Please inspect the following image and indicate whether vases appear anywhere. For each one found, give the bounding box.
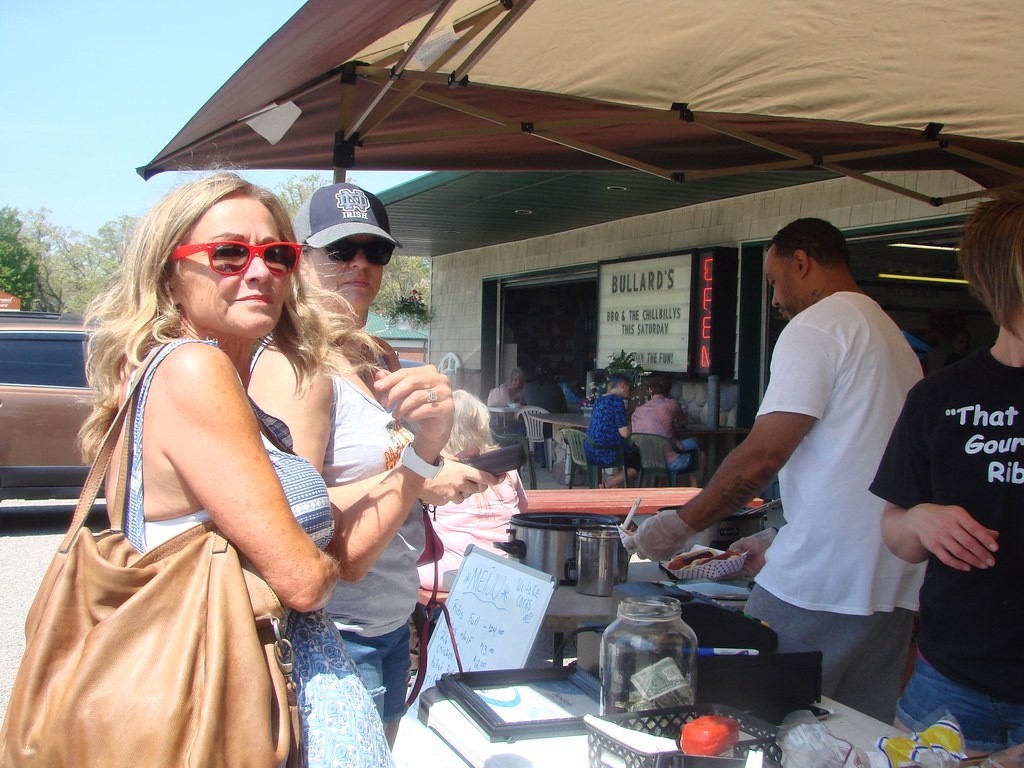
[581,407,593,417]
[397,314,419,330]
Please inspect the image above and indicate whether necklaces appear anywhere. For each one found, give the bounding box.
[330,339,369,381]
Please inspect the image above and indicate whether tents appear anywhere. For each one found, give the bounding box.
[133,0,1024,207]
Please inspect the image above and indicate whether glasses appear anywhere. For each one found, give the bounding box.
[168,241,303,276]
[324,238,395,265]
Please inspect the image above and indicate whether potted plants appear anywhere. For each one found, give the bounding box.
[603,349,651,410]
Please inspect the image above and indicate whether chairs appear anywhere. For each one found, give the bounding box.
[513,406,563,472]
[625,434,702,488]
[490,426,536,490]
[560,428,629,490]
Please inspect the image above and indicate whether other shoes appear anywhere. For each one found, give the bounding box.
[540,461,553,468]
[598,483,605,489]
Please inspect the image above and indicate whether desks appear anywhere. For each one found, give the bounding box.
[529,412,750,487]
[485,406,522,433]
[522,487,763,516]
[545,561,761,668]
[418,669,980,768]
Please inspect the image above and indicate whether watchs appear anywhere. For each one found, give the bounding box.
[401,441,445,479]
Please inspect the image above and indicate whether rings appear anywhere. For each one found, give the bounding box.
[423,387,437,402]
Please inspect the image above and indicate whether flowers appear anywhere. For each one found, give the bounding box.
[581,384,605,407]
[388,288,434,328]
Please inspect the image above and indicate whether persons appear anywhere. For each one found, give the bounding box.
[1,169,455,768]
[867,192,1024,757]
[245,185,507,753]
[584,372,641,490]
[631,371,706,488]
[489,367,567,466]
[623,217,925,729]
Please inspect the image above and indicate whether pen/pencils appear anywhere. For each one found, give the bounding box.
[683,646,759,655]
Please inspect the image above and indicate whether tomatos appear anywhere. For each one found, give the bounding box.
[680,715,739,756]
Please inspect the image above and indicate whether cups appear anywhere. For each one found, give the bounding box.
[576,525,620,595]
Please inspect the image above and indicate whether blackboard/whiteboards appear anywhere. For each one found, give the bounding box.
[405,542,560,713]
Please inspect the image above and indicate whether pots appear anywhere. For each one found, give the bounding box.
[657,503,767,552]
[493,512,630,585]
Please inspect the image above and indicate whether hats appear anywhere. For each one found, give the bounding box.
[293,182,403,249]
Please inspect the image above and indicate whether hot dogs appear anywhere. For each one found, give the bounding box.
[669,548,743,569]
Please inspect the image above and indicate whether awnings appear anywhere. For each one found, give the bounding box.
[371,166,869,256]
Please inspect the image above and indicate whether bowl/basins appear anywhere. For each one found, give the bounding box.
[508,403,520,408]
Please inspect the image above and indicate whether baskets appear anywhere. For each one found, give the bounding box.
[583,702,783,768]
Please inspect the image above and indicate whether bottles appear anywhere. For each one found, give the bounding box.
[680,716,739,759]
[599,596,697,716]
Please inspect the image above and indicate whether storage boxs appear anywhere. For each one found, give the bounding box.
[583,703,782,768]
[661,545,750,578]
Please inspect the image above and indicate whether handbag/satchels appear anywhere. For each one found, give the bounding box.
[415,498,444,567]
[0,340,302,768]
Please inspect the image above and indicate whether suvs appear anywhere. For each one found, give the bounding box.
[0,311,107,513]
